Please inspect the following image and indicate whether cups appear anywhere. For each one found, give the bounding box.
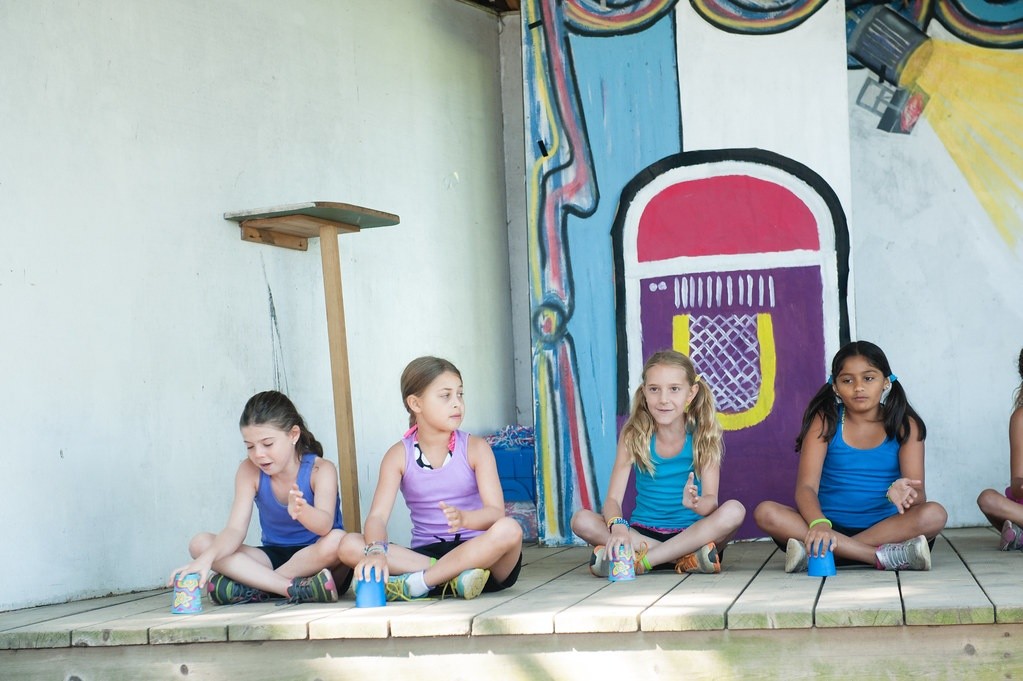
[172,576,203,613]
[355,567,386,608]
[608,546,635,581]
[806,540,836,577]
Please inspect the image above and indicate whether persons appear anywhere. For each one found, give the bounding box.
[753,341,948,572]
[571,350,746,577]
[336,355,523,601]
[977,346,1023,551]
[169,389,354,605]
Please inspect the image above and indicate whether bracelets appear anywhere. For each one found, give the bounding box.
[364,541,388,555]
[809,518,832,529]
[607,516,629,533]
[885,480,897,503]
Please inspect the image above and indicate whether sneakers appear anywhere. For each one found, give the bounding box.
[275,569,337,603]
[351,569,430,603]
[999,519,1023,552]
[875,536,932,571]
[784,537,809,573]
[676,542,722,574]
[206,570,271,605]
[590,545,649,578]
[447,569,491,600]
